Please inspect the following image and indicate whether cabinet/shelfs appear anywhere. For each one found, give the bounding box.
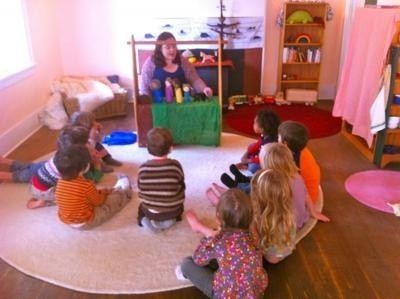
[276,2,328,101]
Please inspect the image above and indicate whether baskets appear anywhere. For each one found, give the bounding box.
[62,90,128,120]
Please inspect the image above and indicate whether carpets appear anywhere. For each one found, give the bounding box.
[223,103,342,139]
[0,127,326,294]
[344,170,400,215]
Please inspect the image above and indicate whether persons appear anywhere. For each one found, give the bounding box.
[204,142,330,231]
[140,31,214,99]
[136,127,186,235]
[93,142,124,174]
[182,166,299,267]
[234,109,282,174]
[50,143,133,232]
[24,124,92,209]
[0,110,105,184]
[174,188,270,299]
[219,119,322,206]
